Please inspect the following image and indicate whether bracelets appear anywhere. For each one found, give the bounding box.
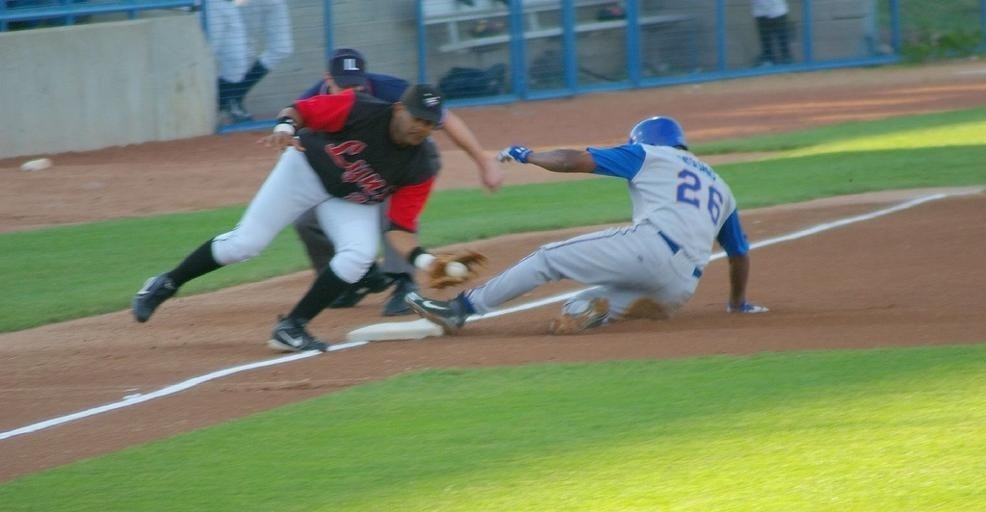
[409,246,426,265]
[272,124,294,136]
[415,254,436,271]
[273,115,296,130]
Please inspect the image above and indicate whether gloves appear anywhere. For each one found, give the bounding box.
[497,143,533,164]
[727,301,771,315]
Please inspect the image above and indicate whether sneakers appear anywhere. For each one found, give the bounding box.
[382,279,419,317]
[269,314,329,354]
[547,295,610,336]
[131,271,179,323]
[222,97,252,123]
[404,290,469,337]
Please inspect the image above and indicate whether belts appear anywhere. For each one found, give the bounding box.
[657,231,704,279]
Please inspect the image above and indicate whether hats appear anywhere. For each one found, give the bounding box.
[328,47,368,89]
[400,83,443,126]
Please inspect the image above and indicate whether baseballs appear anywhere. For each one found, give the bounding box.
[446,261,467,276]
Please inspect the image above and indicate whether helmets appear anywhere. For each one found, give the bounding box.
[628,115,689,151]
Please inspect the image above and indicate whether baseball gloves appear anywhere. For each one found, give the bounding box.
[430,249,486,288]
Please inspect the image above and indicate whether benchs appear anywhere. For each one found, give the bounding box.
[419,1,692,69]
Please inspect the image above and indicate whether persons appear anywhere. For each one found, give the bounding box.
[131,84,443,355]
[749,0,792,69]
[296,47,501,317]
[202,1,293,126]
[405,117,771,338]
